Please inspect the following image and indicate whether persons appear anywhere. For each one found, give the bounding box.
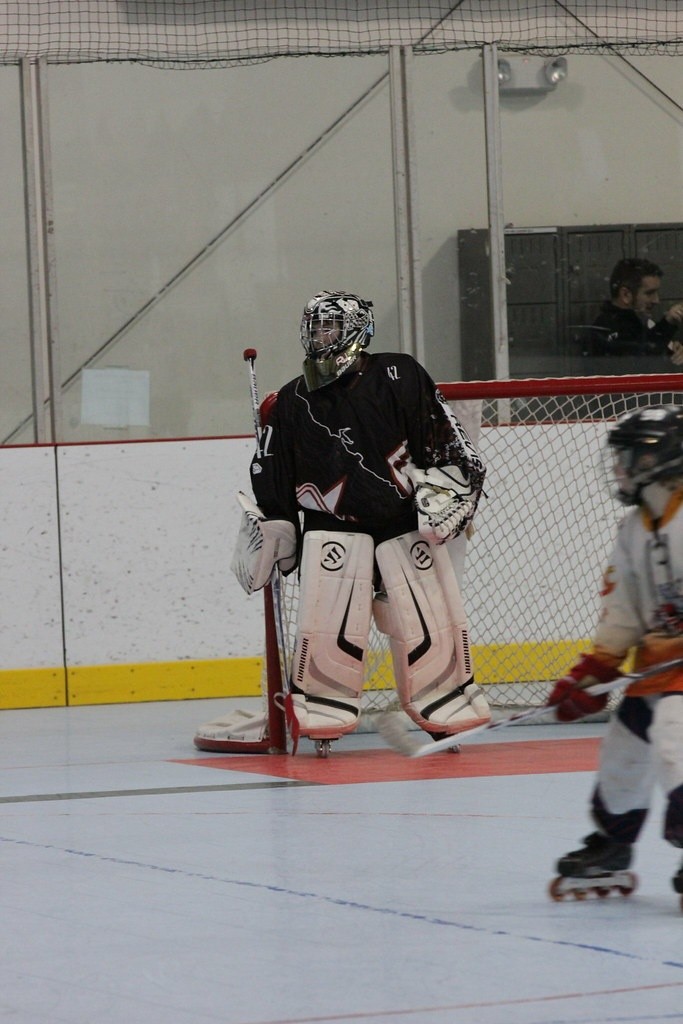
[548,403,683,891]
[578,257,682,375]
[249,290,491,736]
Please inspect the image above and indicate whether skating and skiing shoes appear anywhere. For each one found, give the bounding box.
[428,730,460,754]
[549,834,639,900]
[311,737,335,757]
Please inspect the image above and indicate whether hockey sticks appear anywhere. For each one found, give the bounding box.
[243,348,300,757]
[374,656,683,760]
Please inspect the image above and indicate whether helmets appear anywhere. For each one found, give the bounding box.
[299,290,375,391]
[607,404,683,506]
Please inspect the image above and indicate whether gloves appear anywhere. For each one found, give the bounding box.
[547,653,618,723]
